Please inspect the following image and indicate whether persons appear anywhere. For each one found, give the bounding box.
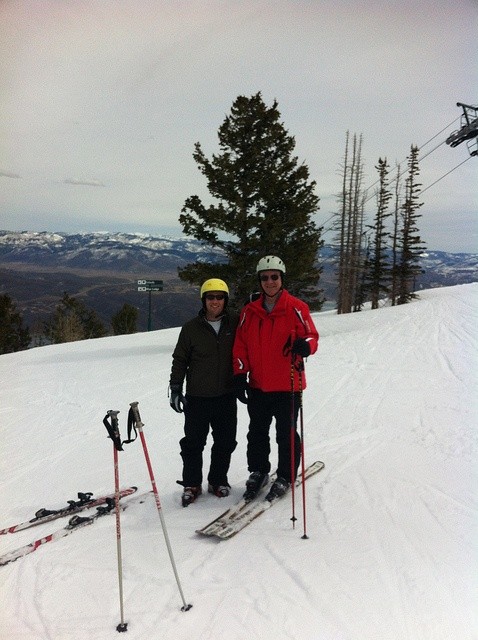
[169,278,245,506]
[233,256,318,494]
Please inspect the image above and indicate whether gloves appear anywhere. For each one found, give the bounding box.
[290,338,312,358]
[232,373,251,403]
[168,384,188,413]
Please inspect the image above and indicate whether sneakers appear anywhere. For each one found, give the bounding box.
[270,478,289,498]
[182,485,201,507]
[246,472,269,491]
[208,482,229,497]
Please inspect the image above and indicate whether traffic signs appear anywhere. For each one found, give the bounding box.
[137,286,163,292]
[137,279,163,285]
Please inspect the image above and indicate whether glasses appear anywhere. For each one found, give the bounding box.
[261,274,278,282]
[205,295,224,300]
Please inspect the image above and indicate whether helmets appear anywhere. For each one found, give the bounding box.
[255,255,286,286]
[200,278,230,300]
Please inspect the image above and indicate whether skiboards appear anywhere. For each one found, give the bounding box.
[195,461,325,541]
[0,486,156,567]
